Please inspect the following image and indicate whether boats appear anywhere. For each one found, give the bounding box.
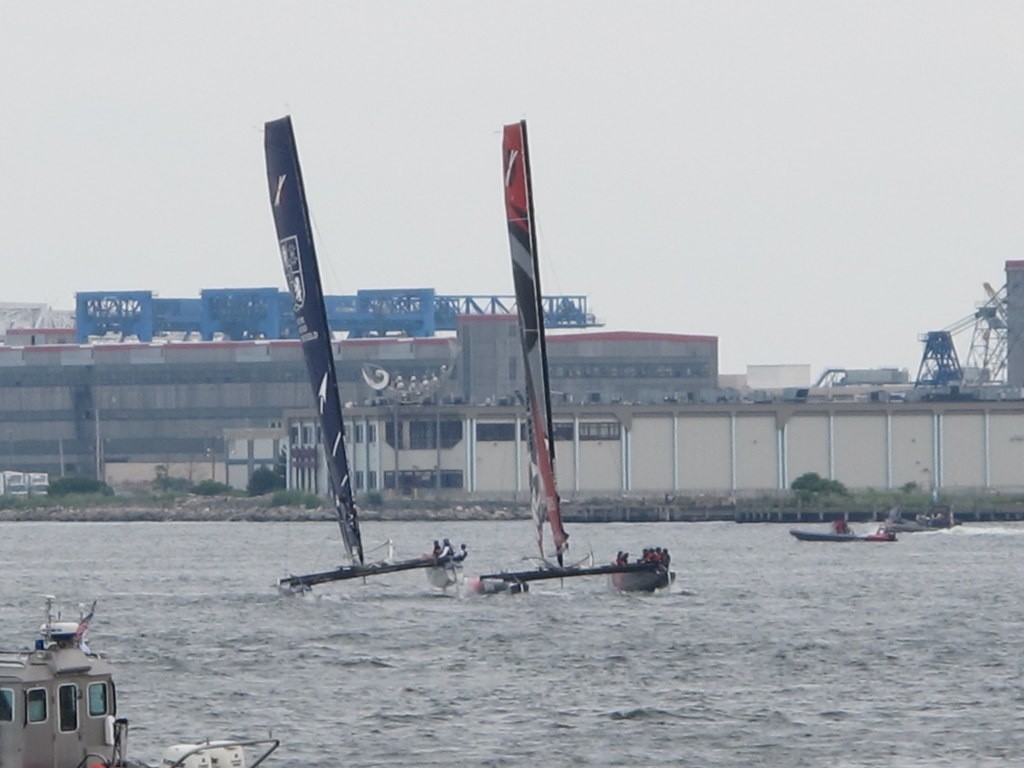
[788,521,899,542]
[0,595,280,768]
[883,504,962,533]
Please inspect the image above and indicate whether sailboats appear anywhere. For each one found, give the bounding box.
[261,116,466,600]
[465,117,678,597]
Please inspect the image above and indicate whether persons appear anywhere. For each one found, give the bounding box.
[918,511,944,521]
[636,548,670,570]
[615,551,629,567]
[832,517,849,534]
[431,538,467,562]
